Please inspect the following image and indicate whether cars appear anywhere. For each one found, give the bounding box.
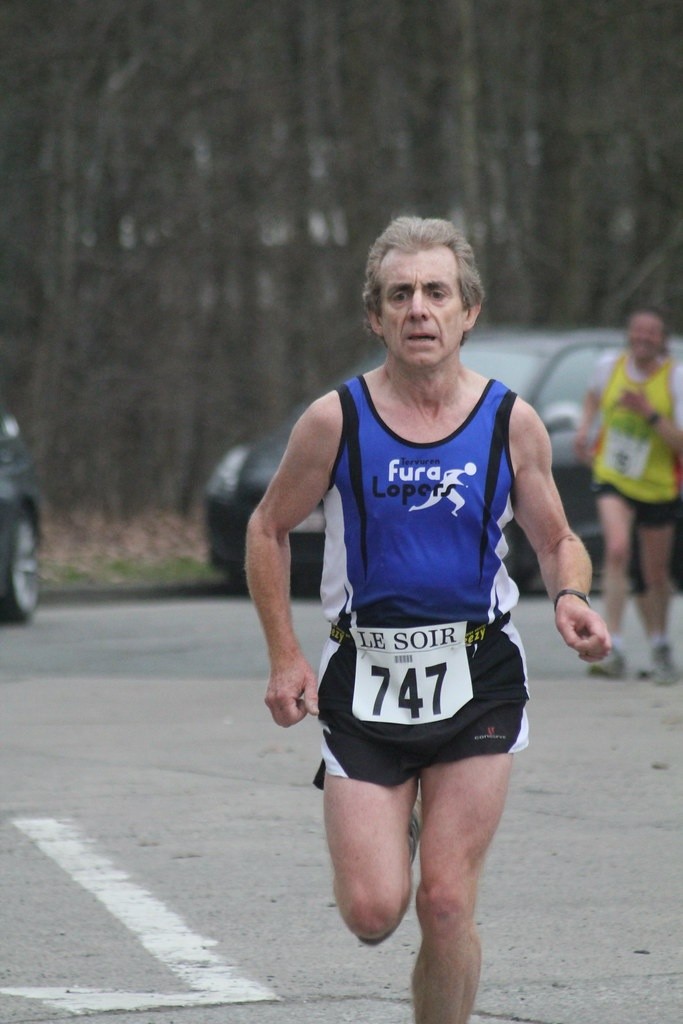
[0,408,43,623]
[200,327,683,607]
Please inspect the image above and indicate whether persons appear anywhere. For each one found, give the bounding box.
[245,212,613,1024]
[575,306,683,684]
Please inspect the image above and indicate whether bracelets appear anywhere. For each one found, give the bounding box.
[647,415,658,425]
[554,589,590,610]
